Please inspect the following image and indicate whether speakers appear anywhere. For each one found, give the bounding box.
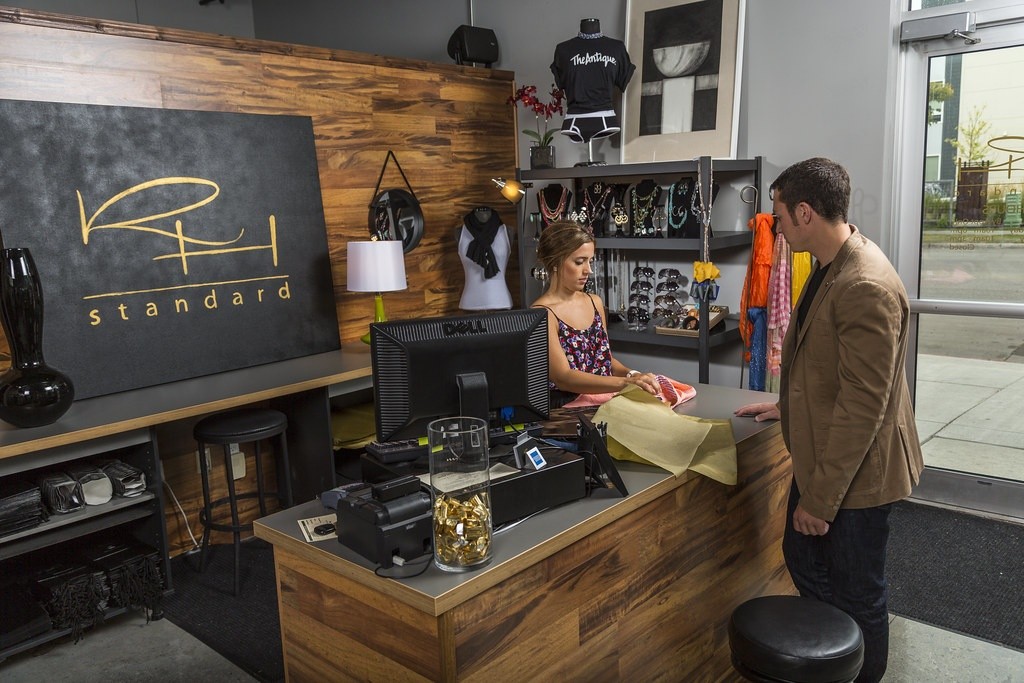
[447,24,498,64]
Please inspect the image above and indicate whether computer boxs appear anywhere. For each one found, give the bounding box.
[360,439,587,525]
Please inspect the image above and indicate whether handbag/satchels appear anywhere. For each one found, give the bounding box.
[0,476,42,537]
[0,532,167,650]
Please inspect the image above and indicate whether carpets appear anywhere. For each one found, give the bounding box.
[152,537,284,683]
[883,499,1023,651]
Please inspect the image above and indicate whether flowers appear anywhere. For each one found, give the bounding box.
[505,83,568,146]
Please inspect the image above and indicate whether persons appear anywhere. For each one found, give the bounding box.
[733,158,925,683]
[453,208,516,311]
[549,17,637,143]
[530,221,659,405]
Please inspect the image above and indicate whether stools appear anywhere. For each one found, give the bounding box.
[192,407,296,599]
[728,594,866,683]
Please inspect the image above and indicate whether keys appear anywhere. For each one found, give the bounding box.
[314,520,337,535]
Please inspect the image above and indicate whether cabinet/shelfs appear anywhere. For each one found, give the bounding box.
[0,349,379,668]
[514,155,763,386]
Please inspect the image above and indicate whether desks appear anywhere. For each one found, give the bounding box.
[253,381,795,683]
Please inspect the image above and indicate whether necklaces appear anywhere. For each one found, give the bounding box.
[691,182,714,218]
[669,184,689,228]
[697,158,714,263]
[584,185,611,231]
[577,31,604,39]
[572,206,586,225]
[631,186,658,237]
[538,185,569,228]
[613,204,628,225]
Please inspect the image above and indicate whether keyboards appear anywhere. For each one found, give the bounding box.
[366,421,544,465]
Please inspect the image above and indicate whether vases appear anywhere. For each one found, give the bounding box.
[530,146,557,169]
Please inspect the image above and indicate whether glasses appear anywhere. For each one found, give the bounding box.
[627,267,680,324]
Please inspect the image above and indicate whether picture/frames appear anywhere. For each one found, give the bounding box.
[620,0,745,160]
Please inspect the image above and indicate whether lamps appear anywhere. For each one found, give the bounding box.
[345,234,408,345]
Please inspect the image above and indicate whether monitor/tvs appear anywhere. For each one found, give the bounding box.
[370,308,550,471]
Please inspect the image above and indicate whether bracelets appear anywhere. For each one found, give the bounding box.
[627,370,641,378]
